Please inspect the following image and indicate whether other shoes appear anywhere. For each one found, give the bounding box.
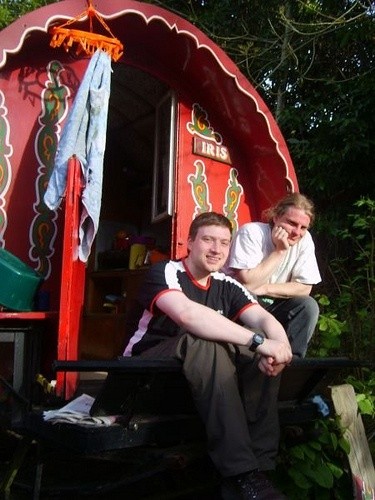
[225,470,285,499]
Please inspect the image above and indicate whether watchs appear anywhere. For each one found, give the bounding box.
[248,332,265,352]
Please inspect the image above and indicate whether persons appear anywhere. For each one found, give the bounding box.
[120,211,293,500]
[217,192,323,360]
